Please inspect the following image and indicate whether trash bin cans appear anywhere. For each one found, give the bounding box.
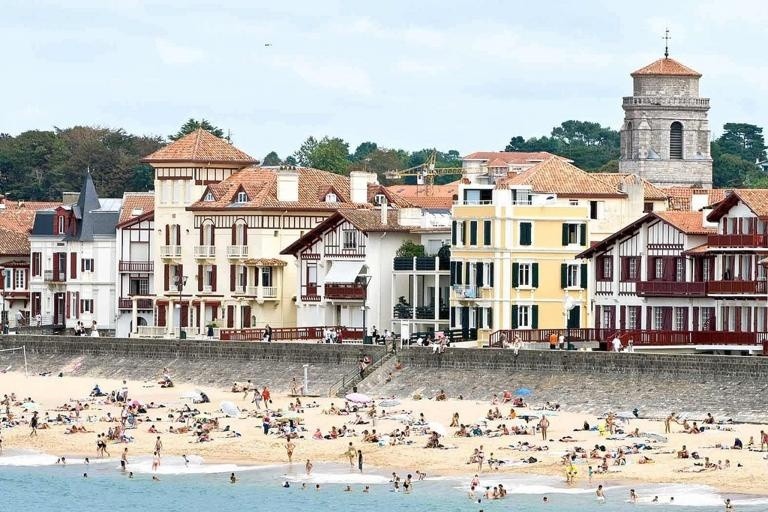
[363,336,372,344]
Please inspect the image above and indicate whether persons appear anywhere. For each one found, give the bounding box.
[611,335,622,352]
[558,332,565,350]
[263,327,269,341]
[550,331,558,350]
[363,354,370,368]
[323,326,343,343]
[3,321,9,334]
[0,366,767,509]
[266,324,272,342]
[393,360,402,370]
[15,310,25,334]
[371,325,396,349]
[417,332,451,355]
[360,360,366,380]
[628,336,633,352]
[36,313,42,326]
[500,331,523,356]
[75,320,99,337]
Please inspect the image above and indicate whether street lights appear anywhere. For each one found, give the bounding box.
[1,269,8,334]
[357,273,372,343]
[173,272,189,339]
[561,295,578,349]
